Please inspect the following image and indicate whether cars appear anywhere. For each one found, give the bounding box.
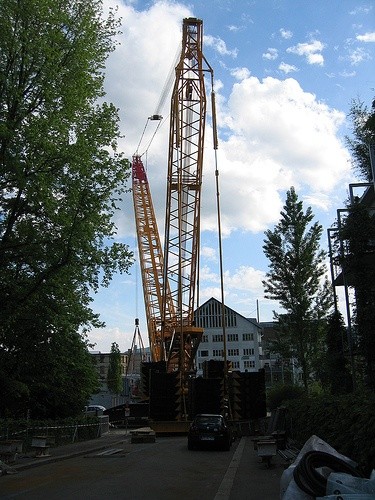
[191,414,232,451]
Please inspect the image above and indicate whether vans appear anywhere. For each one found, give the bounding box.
[81,405,106,416]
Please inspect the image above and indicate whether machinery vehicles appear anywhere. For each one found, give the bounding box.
[125,17,225,402]
